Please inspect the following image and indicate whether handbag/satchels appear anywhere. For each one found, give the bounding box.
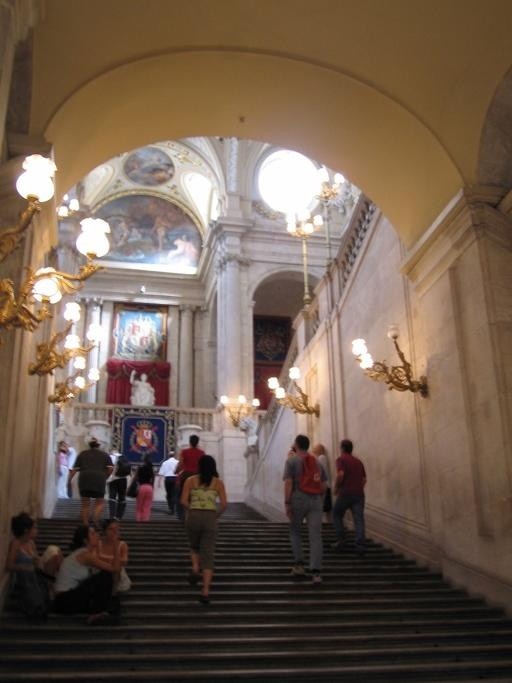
[125,480,139,499]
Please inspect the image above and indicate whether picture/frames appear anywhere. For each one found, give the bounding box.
[113,302,167,362]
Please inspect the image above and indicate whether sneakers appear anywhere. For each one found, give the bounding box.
[351,540,363,550]
[329,537,347,549]
[85,610,111,624]
[193,574,203,584]
[198,594,211,604]
[287,563,305,577]
[312,572,322,583]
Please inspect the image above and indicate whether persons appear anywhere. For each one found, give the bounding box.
[68,437,114,528]
[130,370,155,406]
[114,209,198,264]
[135,457,155,522]
[57,441,78,498]
[180,454,226,604]
[283,434,366,585]
[120,313,158,356]
[157,452,180,515]
[108,448,133,523]
[175,435,206,520]
[4,510,132,620]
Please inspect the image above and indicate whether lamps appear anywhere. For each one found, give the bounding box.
[56,192,80,220]
[48,355,101,404]
[284,171,345,303]
[1,152,112,329]
[29,300,105,378]
[220,394,261,426]
[267,367,320,418]
[350,322,426,394]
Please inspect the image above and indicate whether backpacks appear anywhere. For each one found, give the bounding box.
[115,454,132,476]
[297,454,324,496]
